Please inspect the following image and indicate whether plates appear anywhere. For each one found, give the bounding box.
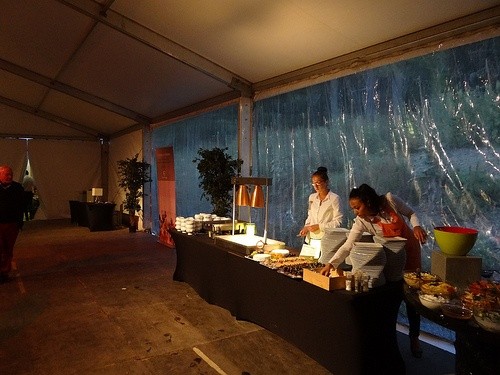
[321,228,407,286]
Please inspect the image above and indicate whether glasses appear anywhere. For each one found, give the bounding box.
[311,180,324,187]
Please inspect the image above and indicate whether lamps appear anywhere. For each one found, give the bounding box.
[91,187,103,203]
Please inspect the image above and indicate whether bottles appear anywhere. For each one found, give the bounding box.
[415,267,421,279]
[346,268,374,293]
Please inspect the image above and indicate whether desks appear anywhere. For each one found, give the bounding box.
[69,200,117,232]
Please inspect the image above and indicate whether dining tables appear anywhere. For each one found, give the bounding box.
[402,276,500,375]
[168,225,404,375]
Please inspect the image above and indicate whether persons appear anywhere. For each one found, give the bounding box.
[22,171,35,223]
[319,184,427,359]
[296,166,342,246]
[0,166,26,282]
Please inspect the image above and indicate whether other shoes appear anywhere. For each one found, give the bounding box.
[410,337,423,358]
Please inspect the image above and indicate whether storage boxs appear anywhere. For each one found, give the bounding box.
[83,189,94,203]
[430,253,483,288]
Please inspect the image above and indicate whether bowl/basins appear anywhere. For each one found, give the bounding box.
[176,213,231,236]
[401,270,500,333]
[434,227,478,256]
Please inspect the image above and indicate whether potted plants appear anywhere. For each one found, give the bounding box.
[193,145,244,217]
[118,148,153,234]
